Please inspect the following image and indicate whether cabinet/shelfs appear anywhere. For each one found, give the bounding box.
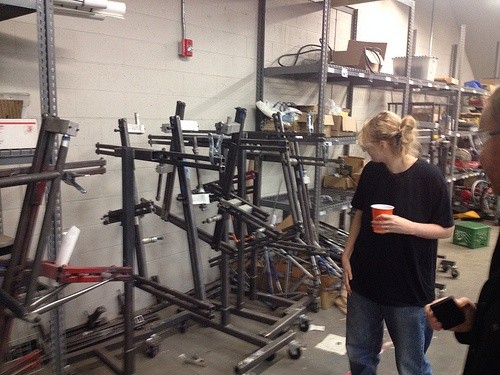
[253,0,500,245]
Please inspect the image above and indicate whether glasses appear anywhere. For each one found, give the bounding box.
[469,131,500,155]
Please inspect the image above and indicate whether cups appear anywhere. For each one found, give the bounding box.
[370,204,396,235]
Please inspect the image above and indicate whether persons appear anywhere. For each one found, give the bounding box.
[423,85,500,375]
[341,110,456,375]
